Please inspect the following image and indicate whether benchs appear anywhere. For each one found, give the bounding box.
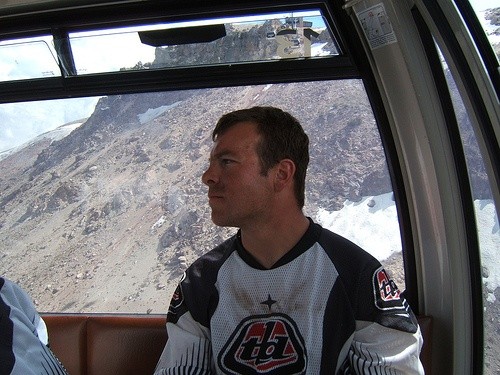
[39,312,433,375]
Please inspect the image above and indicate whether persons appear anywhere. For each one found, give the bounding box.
[151,105,429,375]
[0,277,69,374]
